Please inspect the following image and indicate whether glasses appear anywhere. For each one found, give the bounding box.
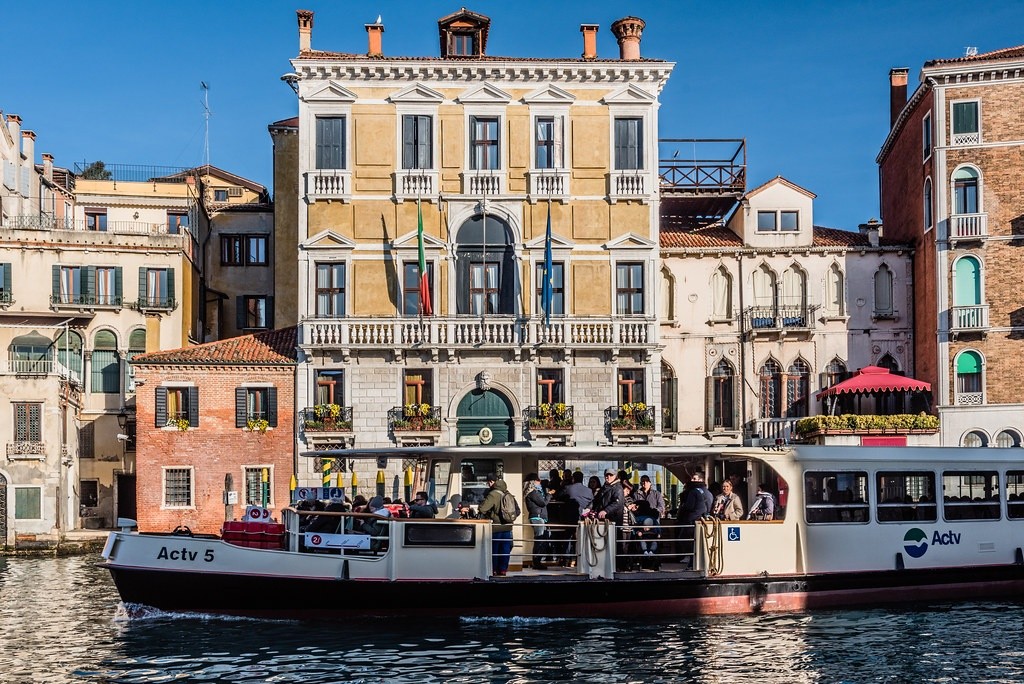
[416,498,425,502]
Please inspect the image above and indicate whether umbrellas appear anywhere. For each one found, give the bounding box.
[816,365,932,417]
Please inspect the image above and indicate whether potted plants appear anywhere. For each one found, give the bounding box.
[612,419,654,429]
[305,422,352,431]
[394,419,441,431]
[526,420,573,430]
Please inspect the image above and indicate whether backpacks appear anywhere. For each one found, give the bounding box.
[493,489,520,523]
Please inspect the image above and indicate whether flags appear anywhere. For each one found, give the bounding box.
[541,215,554,328]
[417,194,432,314]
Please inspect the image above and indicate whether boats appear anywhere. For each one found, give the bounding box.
[93,444,1024,626]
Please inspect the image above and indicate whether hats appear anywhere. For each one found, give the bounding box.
[307,492,315,500]
[483,473,497,481]
[454,501,470,511]
[605,469,618,476]
[371,496,384,508]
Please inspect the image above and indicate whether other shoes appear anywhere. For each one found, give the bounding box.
[533,563,548,570]
[493,569,506,576]
[568,560,575,568]
[644,551,653,555]
[680,556,690,562]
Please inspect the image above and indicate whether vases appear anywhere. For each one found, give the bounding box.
[405,411,421,417]
[328,410,332,416]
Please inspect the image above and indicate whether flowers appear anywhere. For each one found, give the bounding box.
[177,419,189,431]
[540,403,565,416]
[403,401,432,415]
[312,402,340,417]
[247,419,269,434]
[622,402,646,414]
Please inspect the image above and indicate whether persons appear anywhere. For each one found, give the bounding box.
[621,479,638,573]
[944,494,1000,520]
[677,478,707,562]
[880,496,937,521]
[454,500,476,519]
[1008,492,1024,519]
[539,468,628,567]
[479,370,492,390]
[631,475,666,555]
[805,479,869,523]
[746,484,774,521]
[691,472,714,515]
[478,473,510,577]
[713,480,743,521]
[296,491,440,550]
[589,468,625,572]
[523,473,556,569]
